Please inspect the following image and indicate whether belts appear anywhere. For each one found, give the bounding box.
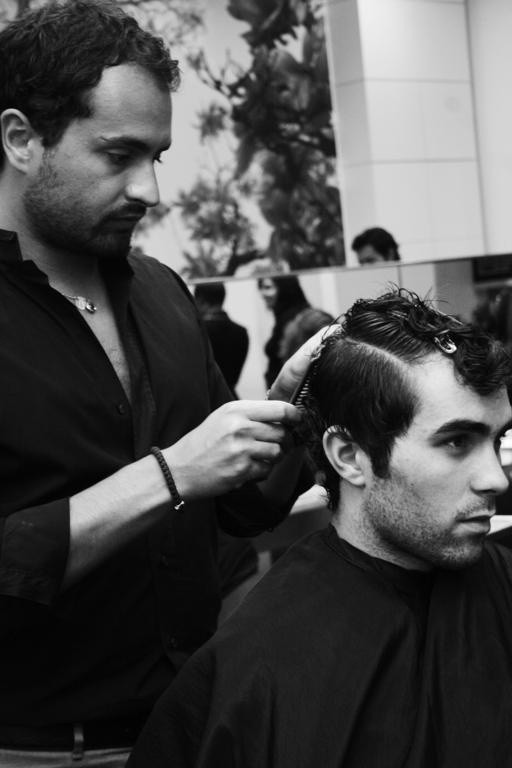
[0,720,149,752]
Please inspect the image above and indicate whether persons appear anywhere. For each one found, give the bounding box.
[0,0,338,768]
[127,286,512,767]
[194,281,251,392]
[350,226,400,264]
[258,275,312,392]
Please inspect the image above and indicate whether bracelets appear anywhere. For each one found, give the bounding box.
[151,446,186,514]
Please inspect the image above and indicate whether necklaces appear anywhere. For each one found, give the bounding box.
[61,294,100,317]
